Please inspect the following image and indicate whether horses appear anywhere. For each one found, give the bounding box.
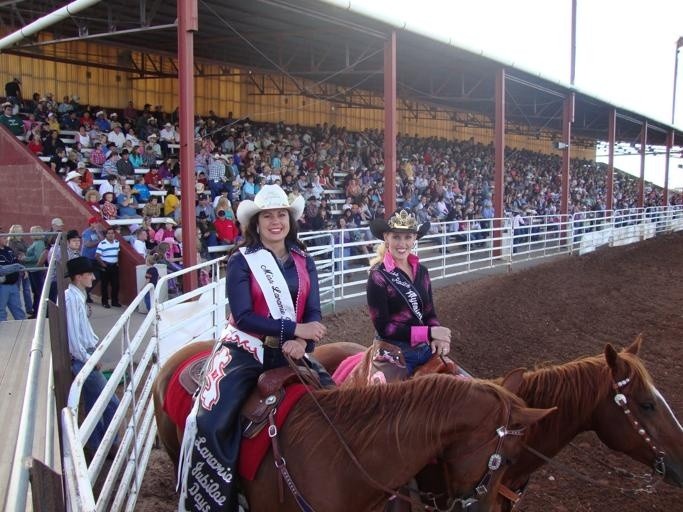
[151,338,559,512]
[309,331,682,511]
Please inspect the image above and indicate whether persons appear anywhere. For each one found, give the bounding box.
[195,110,384,276]
[570,156,683,233]
[396,133,495,251]
[503,145,562,252]
[53,256,120,468]
[185,182,337,512]
[2,76,183,321]
[365,206,452,387]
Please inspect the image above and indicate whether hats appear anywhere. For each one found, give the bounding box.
[85,190,100,203]
[107,142,130,156]
[163,218,177,227]
[96,111,122,127]
[128,224,142,234]
[198,193,207,200]
[51,218,64,226]
[72,96,80,103]
[89,216,99,223]
[64,256,98,277]
[67,230,81,240]
[144,103,171,141]
[196,211,209,219]
[304,183,316,201]
[284,171,305,176]
[370,207,431,240]
[216,154,230,163]
[39,93,54,117]
[14,76,22,84]
[64,161,85,183]
[236,184,305,226]
[2,102,14,107]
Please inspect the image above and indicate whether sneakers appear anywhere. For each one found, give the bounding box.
[87,296,121,308]
[27,311,35,319]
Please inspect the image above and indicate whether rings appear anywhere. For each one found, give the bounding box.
[442,348,448,351]
